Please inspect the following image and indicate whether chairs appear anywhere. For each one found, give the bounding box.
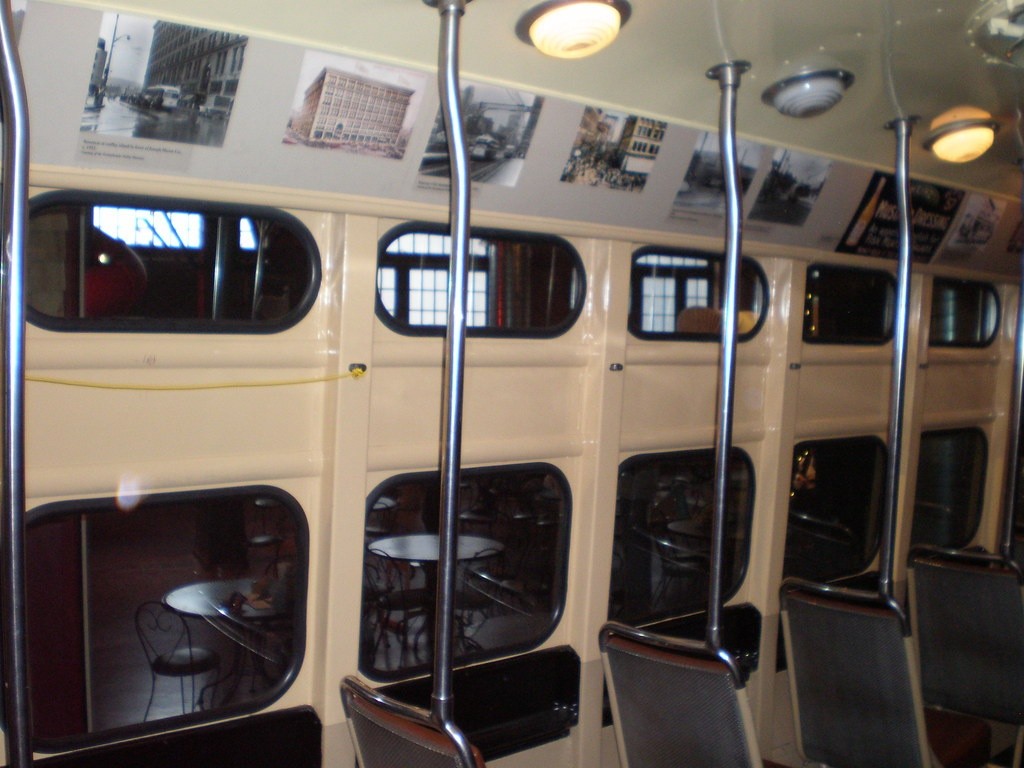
[593,623,761,768]
[777,577,931,768]
[240,477,734,675]
[132,600,219,720]
[344,677,485,768]
[906,543,1024,768]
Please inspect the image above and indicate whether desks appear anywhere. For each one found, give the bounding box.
[365,531,504,561]
[666,517,793,543]
[451,503,547,522]
[159,580,292,621]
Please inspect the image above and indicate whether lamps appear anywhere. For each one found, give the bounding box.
[760,68,854,119]
[514,0,632,58]
[921,107,1000,163]
